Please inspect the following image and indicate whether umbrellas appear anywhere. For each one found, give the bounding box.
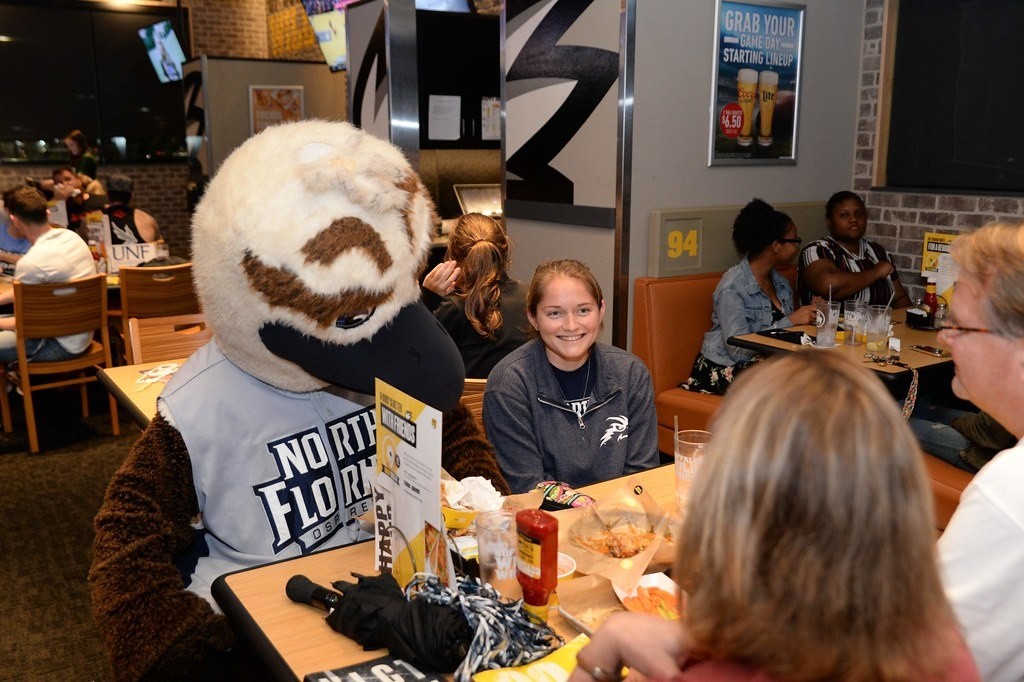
[286,566,476,675]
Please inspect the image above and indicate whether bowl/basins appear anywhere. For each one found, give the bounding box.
[569,511,682,570]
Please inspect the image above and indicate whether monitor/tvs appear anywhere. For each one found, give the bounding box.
[136,18,187,85]
[301,0,478,73]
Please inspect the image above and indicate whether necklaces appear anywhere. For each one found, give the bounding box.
[551,352,591,412]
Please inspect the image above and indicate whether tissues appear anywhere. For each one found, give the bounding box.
[440,475,509,529]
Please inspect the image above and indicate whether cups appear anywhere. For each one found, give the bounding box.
[843,300,869,347]
[475,510,520,604]
[866,305,893,353]
[548,551,577,609]
[674,431,712,516]
[757,71,778,146]
[737,69,758,146]
[816,301,841,346]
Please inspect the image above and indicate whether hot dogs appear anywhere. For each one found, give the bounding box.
[624,586,681,621]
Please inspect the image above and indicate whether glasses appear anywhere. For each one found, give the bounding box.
[925,295,1004,334]
[769,236,803,249]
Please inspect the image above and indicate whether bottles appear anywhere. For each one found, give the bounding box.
[89,240,107,273]
[516,510,559,624]
[916,278,947,329]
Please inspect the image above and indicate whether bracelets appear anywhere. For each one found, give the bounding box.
[575,652,619,682]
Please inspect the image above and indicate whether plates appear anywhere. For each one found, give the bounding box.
[610,572,679,611]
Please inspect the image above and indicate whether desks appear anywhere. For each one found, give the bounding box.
[727,305,955,385]
[209,461,676,682]
[0,257,189,436]
[95,356,189,429]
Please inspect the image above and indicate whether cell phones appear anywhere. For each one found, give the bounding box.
[909,346,952,357]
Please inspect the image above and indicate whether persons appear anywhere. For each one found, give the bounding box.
[932,218,1024,682]
[481,259,661,496]
[27,127,163,272]
[678,194,828,397]
[420,211,541,379]
[565,344,982,682]
[795,187,913,310]
[895,395,1018,473]
[0,185,99,367]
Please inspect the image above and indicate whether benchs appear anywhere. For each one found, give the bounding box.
[920,449,976,537]
[632,265,801,457]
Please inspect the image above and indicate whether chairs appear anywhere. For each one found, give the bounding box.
[0,262,211,456]
[459,378,486,438]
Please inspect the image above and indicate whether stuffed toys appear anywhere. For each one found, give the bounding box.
[88,116,509,682]
